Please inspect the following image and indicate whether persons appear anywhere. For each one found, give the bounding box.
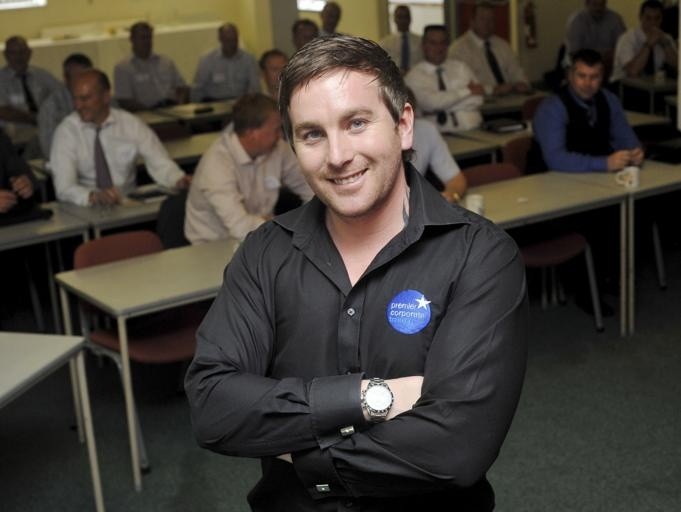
[182,34,530,512]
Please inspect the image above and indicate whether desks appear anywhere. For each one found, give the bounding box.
[1,331,103,512]
[0,96,244,494]
[442,89,681,337]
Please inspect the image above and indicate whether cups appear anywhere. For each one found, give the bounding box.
[466,194,486,217]
[656,71,666,85]
[615,167,641,189]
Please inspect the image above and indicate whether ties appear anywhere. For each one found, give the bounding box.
[95,128,114,189]
[438,68,447,124]
[402,33,410,71]
[485,40,505,85]
[17,74,39,114]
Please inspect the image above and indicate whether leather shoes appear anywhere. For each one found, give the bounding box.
[575,293,614,317]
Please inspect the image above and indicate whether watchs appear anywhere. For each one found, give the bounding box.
[361,377,394,424]
